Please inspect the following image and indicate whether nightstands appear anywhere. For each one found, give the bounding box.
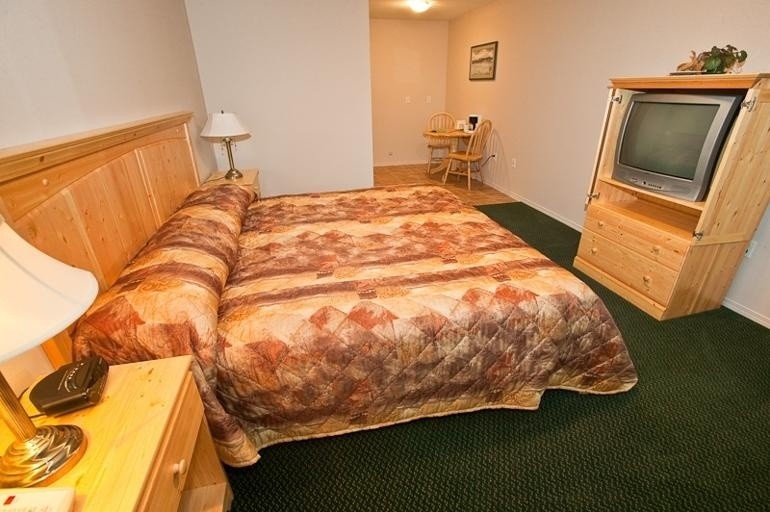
[206,169,261,201]
[0,354,235,512]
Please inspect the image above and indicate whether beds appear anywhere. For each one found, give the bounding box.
[0,112,639,468]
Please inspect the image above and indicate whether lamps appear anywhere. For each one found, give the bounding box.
[199,110,252,179]
[0,214,101,489]
[406,1,434,14]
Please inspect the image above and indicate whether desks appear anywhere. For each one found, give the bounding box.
[425,129,484,182]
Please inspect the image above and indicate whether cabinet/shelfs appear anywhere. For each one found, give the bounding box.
[572,73,769,321]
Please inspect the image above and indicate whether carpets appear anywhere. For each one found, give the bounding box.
[224,202,770,512]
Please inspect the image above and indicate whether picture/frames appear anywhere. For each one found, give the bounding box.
[469,41,497,80]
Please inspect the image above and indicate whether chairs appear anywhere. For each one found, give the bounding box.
[426,112,457,175]
[444,119,492,190]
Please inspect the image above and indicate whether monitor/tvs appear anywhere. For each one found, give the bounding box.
[611,93,745,203]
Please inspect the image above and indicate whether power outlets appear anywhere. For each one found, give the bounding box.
[492,153,496,160]
[745,239,757,257]
